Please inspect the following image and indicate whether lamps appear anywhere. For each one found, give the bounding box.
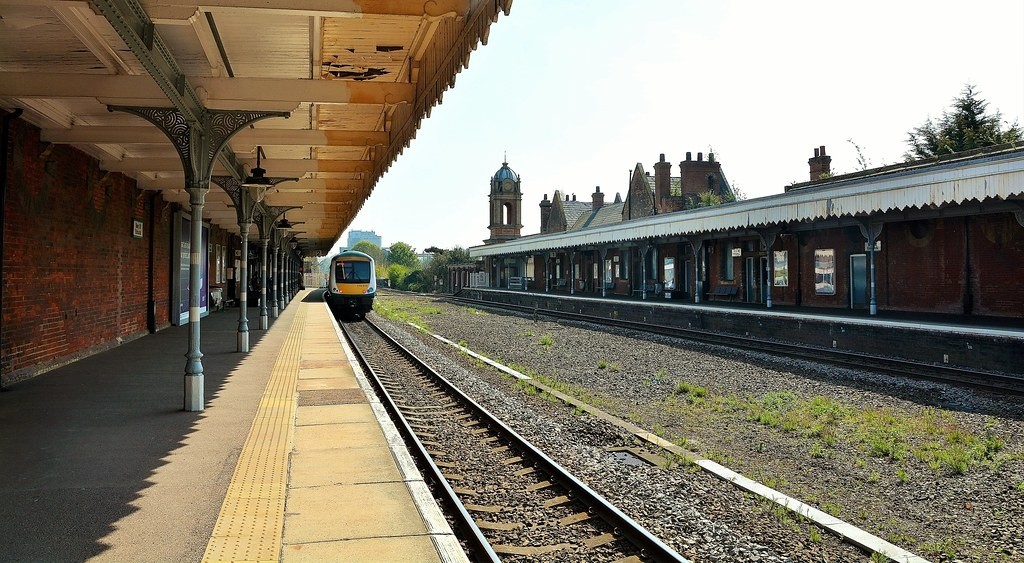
[240,146,275,203]
[288,238,298,249]
[276,212,293,237]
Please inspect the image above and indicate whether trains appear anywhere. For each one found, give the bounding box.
[327,249,376,310]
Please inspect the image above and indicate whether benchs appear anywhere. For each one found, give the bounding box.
[221,300,235,310]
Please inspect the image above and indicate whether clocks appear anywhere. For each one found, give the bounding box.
[504,182,512,191]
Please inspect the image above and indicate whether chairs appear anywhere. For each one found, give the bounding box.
[551,278,737,303]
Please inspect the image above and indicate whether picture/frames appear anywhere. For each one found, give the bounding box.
[132,218,144,239]
